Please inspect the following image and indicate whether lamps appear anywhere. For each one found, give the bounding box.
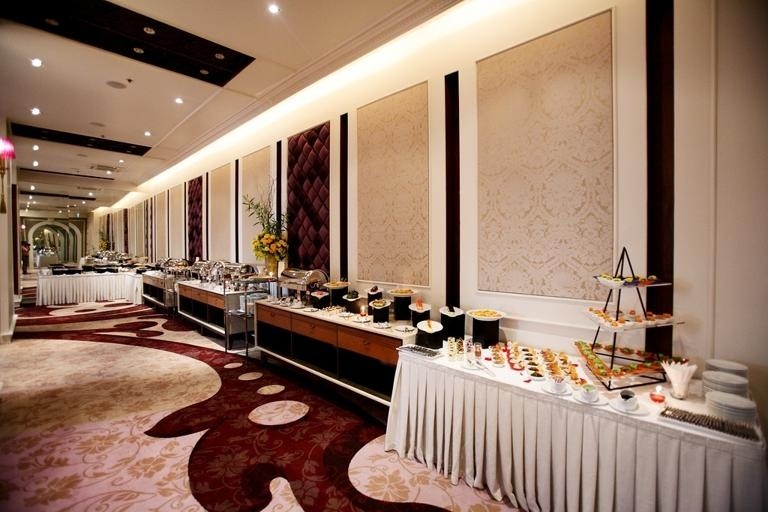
[0,136,16,214]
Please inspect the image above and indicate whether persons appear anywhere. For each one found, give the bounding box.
[20,241,31,274]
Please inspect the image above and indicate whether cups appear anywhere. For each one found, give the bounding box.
[581,384,597,401]
[551,375,565,391]
[617,388,639,410]
[666,374,690,399]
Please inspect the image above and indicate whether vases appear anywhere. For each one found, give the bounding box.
[265,254,278,279]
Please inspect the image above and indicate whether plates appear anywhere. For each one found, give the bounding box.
[609,397,648,415]
[542,382,572,396]
[573,392,610,406]
[522,344,545,381]
[701,358,758,427]
[466,309,504,322]
[237,280,465,334]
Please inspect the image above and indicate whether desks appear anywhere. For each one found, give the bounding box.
[36,247,59,267]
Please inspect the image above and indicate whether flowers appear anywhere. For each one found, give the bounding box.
[98,231,109,251]
[241,178,291,260]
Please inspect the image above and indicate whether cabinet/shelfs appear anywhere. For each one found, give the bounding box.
[93,251,191,311]
[178,280,269,349]
[225,275,281,359]
[254,295,418,408]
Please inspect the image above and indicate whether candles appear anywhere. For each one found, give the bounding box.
[650,385,665,405]
[359,305,366,317]
